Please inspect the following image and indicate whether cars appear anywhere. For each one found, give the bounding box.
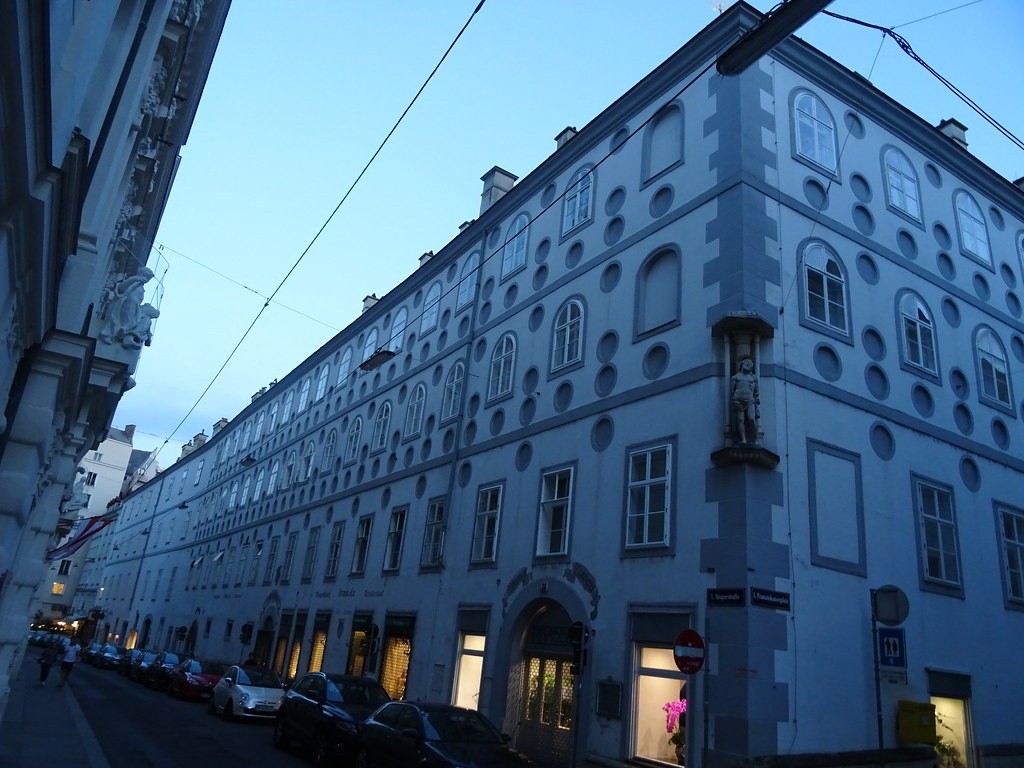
[274,674,537,768]
[28,630,72,647]
[81,642,286,722]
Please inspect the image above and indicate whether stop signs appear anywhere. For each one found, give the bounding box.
[675,630,705,674]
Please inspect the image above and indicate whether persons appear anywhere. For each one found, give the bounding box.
[244,653,256,666]
[56,637,81,687]
[37,637,58,686]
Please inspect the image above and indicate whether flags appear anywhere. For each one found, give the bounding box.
[47,516,114,562]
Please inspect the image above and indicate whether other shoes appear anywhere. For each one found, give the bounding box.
[57,683,64,688]
[41,681,46,686]
